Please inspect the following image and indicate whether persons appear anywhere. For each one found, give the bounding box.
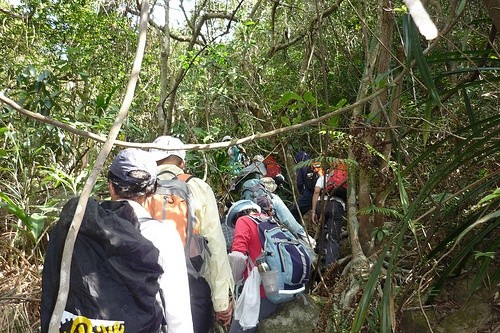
[311,164,348,287]
[40,147,195,333]
[290,151,325,224]
[241,174,317,250]
[222,136,251,188]
[229,154,285,194]
[141,136,236,333]
[221,200,312,333]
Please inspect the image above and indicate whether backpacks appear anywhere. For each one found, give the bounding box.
[241,179,273,215]
[302,163,321,192]
[141,169,213,280]
[246,214,311,304]
[319,161,349,201]
[256,154,285,186]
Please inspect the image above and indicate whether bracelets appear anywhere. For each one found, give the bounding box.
[228,293,234,302]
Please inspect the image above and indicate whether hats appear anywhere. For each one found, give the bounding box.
[221,135,233,141]
[151,134,187,165]
[110,147,157,188]
[225,200,262,230]
[252,154,265,163]
